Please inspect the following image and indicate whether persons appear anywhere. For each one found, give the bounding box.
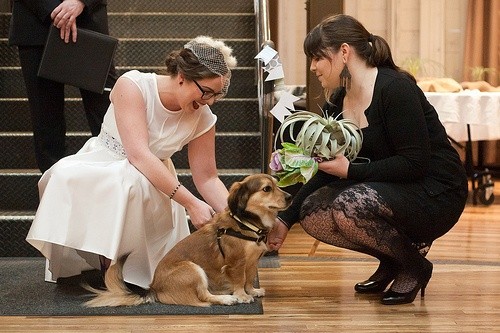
[7,0,118,175]
[25,34,238,290]
[264,14,468,305]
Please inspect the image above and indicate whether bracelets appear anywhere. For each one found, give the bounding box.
[170,183,181,200]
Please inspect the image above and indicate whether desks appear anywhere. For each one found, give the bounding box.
[424,91,500,205]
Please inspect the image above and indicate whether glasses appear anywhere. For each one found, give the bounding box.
[194,79,223,100]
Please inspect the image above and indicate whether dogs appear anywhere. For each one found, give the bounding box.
[77,174,293,307]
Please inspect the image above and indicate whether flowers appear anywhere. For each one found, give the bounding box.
[269,104,364,188]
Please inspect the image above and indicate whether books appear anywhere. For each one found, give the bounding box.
[36,23,118,96]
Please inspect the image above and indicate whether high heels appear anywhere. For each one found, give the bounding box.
[380,258,433,305]
[354,261,394,294]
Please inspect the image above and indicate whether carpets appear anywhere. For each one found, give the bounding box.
[0,257,264,317]
